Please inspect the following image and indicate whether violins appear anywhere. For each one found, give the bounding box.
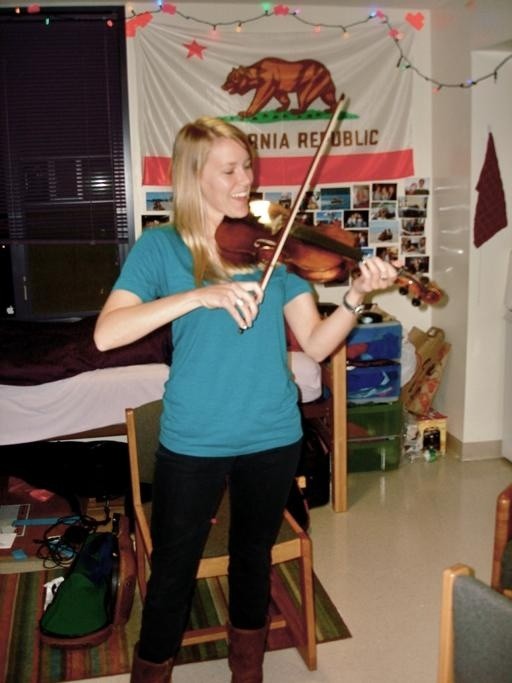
[215,198,448,307]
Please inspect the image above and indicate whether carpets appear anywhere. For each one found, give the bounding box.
[0,494,353,681]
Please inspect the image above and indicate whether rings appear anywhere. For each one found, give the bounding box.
[379,276,388,281]
[234,300,251,309]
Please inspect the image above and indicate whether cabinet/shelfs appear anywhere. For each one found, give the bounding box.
[282,299,349,514]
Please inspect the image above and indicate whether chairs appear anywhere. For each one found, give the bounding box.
[491,484,512,598]
[439,559,511,683]
[122,394,323,674]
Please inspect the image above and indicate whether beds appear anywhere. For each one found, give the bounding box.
[0,312,174,505]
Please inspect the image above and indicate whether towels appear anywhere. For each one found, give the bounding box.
[473,128,508,249]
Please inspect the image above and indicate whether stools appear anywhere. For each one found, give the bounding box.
[405,407,451,458]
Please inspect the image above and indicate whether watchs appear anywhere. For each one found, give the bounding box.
[342,292,366,316]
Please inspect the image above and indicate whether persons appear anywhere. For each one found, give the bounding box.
[93,117,399,682]
[153,200,165,210]
[298,177,430,272]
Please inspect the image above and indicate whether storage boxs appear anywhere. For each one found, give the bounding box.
[340,306,405,475]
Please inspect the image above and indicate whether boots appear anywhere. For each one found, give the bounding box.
[225,613,273,683]
[130,639,175,683]
